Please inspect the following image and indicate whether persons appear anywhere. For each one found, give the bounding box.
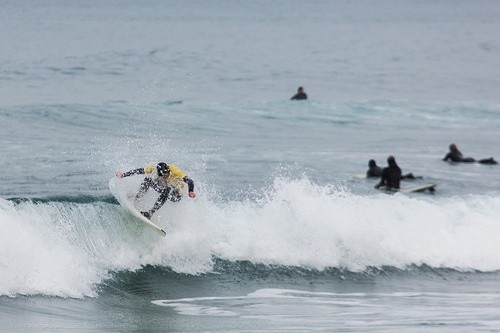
[292,87,307,100]
[375,155,402,189]
[366,159,423,180]
[443,144,462,162]
[116,162,196,219]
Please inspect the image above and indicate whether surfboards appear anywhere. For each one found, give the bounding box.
[107,174,168,237]
[405,180,432,192]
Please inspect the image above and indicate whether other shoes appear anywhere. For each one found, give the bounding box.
[140,211,151,220]
